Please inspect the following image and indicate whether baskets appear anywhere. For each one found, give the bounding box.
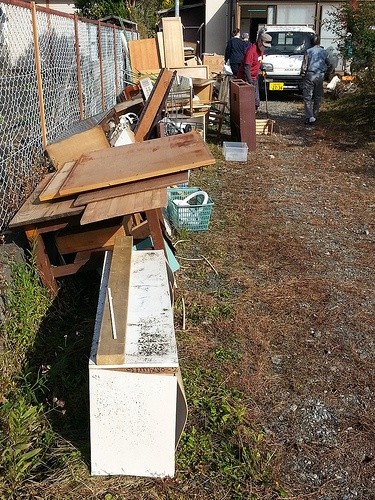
[166,187,215,231]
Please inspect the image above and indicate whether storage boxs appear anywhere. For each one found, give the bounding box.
[167,187,214,231]
[223,142,249,161]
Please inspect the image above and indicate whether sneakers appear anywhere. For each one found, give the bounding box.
[306,117,315,125]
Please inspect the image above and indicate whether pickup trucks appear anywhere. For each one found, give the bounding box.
[258,25,316,90]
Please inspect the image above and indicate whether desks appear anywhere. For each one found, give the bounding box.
[45,106,120,170]
[9,171,169,298]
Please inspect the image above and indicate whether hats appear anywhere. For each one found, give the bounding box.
[260,34,272,48]
[241,33,249,38]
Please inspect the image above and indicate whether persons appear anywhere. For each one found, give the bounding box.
[344,33,354,76]
[300,34,334,125]
[225,28,272,115]
